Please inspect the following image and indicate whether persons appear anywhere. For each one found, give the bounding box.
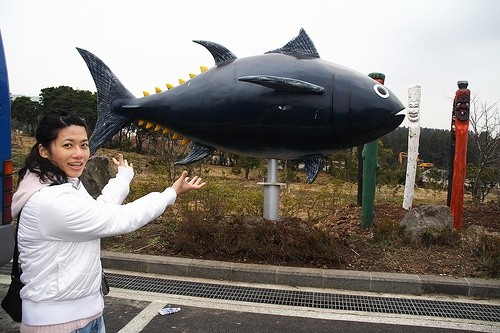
[0,109,206,333]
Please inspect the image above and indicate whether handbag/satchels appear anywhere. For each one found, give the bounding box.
[1,271,25,322]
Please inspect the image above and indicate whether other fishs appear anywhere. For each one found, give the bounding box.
[75,27,406,186]
